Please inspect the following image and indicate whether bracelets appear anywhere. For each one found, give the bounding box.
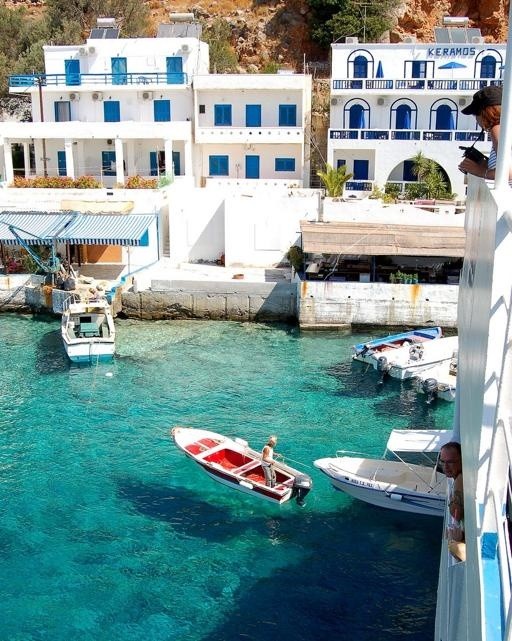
[485,169,488,179]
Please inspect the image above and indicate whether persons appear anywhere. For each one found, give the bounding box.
[440,442,466,561]
[261,436,281,488]
[458,85,512,188]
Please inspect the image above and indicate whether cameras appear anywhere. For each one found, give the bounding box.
[458,146,489,175]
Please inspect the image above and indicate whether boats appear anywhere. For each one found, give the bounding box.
[60,292,117,365]
[169,425,313,508]
[352,324,458,402]
[311,426,458,517]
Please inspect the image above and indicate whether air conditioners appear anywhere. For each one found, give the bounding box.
[331,98,342,106]
[78,47,87,56]
[472,37,484,43]
[69,93,79,100]
[87,46,96,55]
[459,98,471,106]
[92,91,102,100]
[376,98,390,107]
[143,92,152,99]
[405,37,416,43]
[106,139,115,146]
[346,37,358,44]
[181,44,191,52]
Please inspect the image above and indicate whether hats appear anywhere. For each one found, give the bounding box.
[462,87,500,114]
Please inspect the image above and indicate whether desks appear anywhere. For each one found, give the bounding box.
[0,264,8,272]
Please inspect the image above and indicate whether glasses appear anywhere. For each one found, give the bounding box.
[439,458,459,465]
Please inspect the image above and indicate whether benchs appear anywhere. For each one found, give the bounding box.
[233,460,261,475]
[384,343,400,348]
